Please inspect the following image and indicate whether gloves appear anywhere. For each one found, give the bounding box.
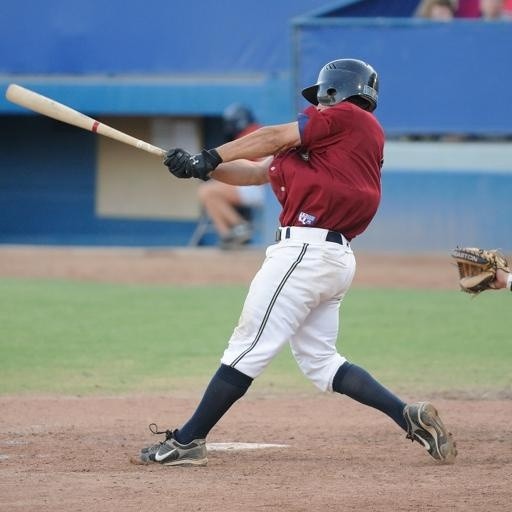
[163,146,193,179]
[184,148,223,182]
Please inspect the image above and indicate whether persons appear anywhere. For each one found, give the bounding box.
[197,103,272,249]
[479,1,504,21]
[485,264,512,293]
[132,57,459,468]
[416,1,455,18]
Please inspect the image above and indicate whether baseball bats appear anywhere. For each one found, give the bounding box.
[6,83,213,178]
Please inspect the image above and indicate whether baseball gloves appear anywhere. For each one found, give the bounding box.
[451,246,508,292]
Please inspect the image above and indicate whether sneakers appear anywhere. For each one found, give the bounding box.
[131,427,209,467]
[403,401,458,465]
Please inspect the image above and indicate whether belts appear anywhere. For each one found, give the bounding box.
[275,228,343,246]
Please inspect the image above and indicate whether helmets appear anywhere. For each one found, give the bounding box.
[300,58,378,112]
